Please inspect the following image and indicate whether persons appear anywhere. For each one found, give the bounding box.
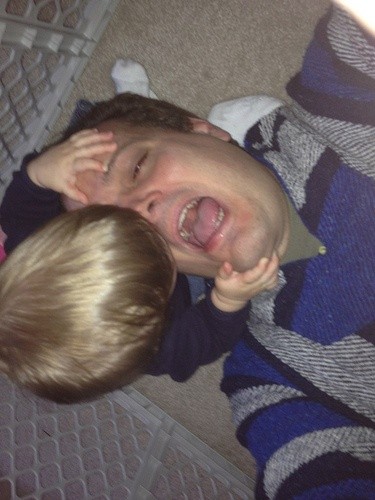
[0,122,282,407]
[63,1,374,500]
